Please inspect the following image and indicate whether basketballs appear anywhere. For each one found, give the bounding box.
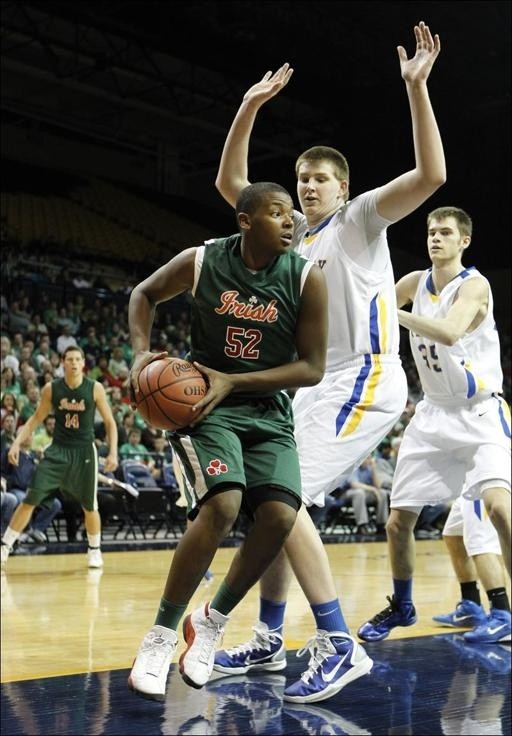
[131,355,206,430]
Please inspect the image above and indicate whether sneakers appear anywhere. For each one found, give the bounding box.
[282,628,373,704]
[87,547,105,568]
[126,623,179,704]
[178,600,226,690]
[357,593,417,642]
[357,520,444,538]
[431,599,487,627]
[1,540,10,567]
[462,608,512,645]
[213,623,288,676]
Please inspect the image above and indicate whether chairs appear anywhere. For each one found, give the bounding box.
[318,505,378,536]
[0,182,223,268]
[42,458,186,540]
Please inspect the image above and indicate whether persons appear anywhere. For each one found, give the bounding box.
[124,182,328,705]
[431,494,512,644]
[355,206,512,644]
[209,15,446,705]
[1,346,119,567]
[0,254,191,543]
[438,655,508,736]
[316,345,442,542]
